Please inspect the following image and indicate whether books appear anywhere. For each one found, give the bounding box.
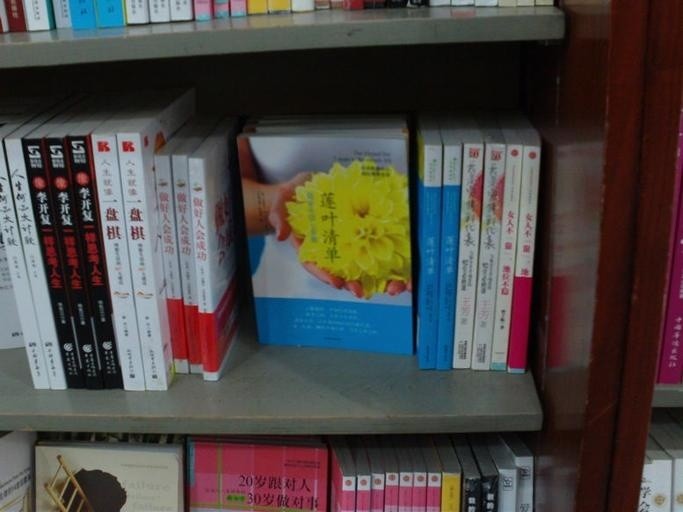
[0,65,543,394]
[655,125,683,384]
[0,410,682,512]
[2,0,559,36]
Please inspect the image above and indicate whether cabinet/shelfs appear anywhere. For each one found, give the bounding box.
[1,1,614,511]
